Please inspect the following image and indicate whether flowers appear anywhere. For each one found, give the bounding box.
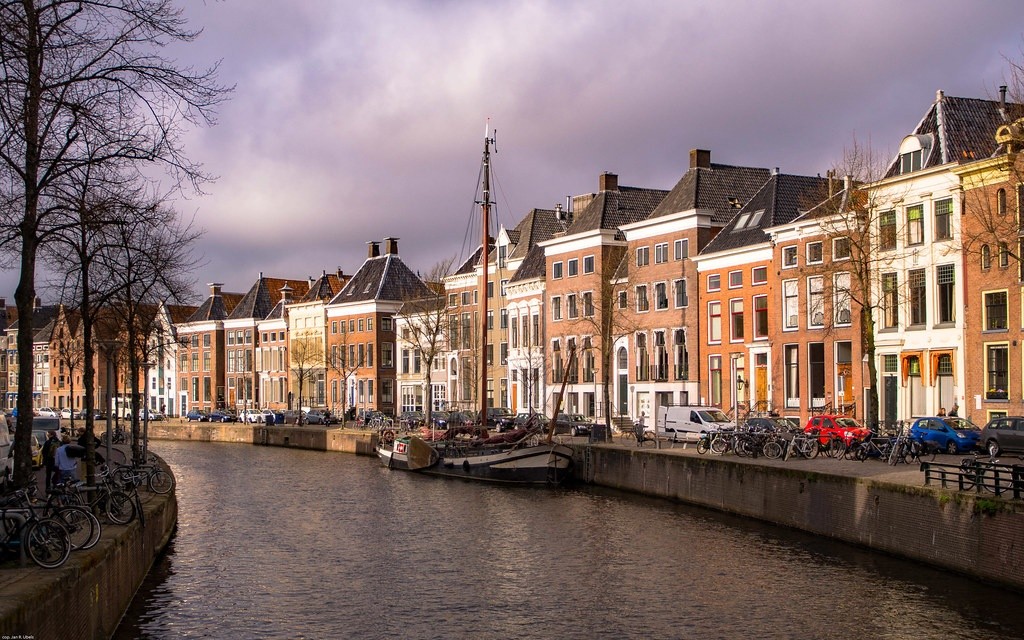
[989,388,1004,393]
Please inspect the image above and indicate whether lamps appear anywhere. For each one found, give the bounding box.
[737,374,749,390]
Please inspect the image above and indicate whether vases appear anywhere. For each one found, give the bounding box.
[986,391,1007,399]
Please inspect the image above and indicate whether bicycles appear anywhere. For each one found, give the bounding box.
[960,443,1012,494]
[620,424,655,445]
[100,424,131,445]
[697,418,936,467]
[0,456,173,569]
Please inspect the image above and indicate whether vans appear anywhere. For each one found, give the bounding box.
[111,397,132,420]
[658,405,735,445]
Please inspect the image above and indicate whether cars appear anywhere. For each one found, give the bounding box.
[0,412,61,484]
[804,415,871,450]
[910,414,1024,457]
[401,407,606,437]
[356,409,392,425]
[186,409,338,425]
[744,417,803,433]
[39,407,164,421]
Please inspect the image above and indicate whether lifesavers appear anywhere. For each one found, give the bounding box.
[396,444,403,452]
[384,431,395,442]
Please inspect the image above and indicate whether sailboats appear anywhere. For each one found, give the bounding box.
[375,113,573,485]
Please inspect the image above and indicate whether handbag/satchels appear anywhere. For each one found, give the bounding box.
[65,444,86,457]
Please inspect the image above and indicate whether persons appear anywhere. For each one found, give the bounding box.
[636,411,645,438]
[937,408,946,416]
[77,428,101,481]
[948,404,959,423]
[324,408,331,422]
[771,409,780,417]
[161,404,166,413]
[59,427,68,442]
[54,437,86,504]
[41,430,61,495]
[7,427,16,459]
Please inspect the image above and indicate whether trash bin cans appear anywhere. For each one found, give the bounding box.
[265,415,273,425]
[274,413,285,427]
[589,424,607,441]
